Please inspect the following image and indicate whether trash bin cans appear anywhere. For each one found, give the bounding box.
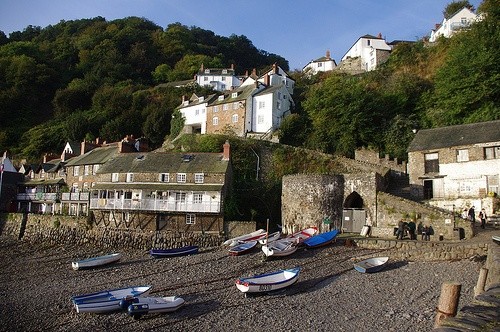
[453,228,460,240]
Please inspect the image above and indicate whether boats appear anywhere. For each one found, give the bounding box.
[235,267,300,293]
[227,240,259,255]
[491,236,500,246]
[261,218,300,257]
[149,245,199,258]
[221,228,281,247]
[71,285,152,313]
[302,229,339,249]
[286,223,318,248]
[71,252,122,271]
[353,256,389,273]
[119,295,186,320]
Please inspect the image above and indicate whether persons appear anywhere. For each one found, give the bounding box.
[468,205,475,222]
[478,208,487,228]
[396,219,405,240]
[421,225,429,241]
[408,219,416,239]
